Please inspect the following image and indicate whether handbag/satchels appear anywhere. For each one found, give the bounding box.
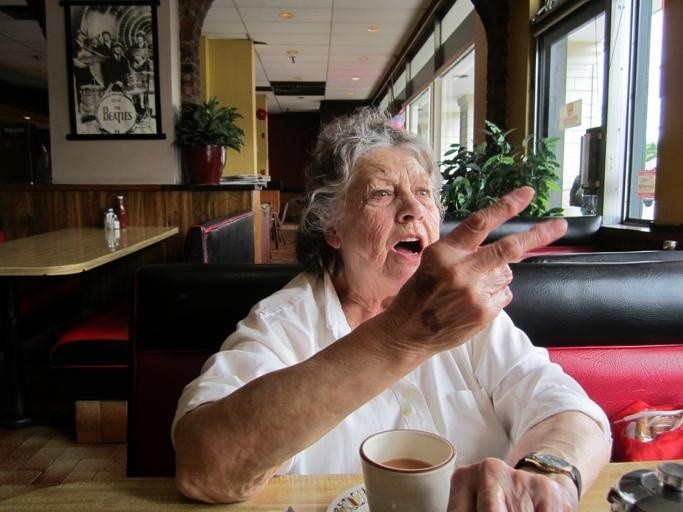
[606,400,683,462]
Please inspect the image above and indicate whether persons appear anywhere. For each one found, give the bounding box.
[69,29,150,115]
[170,106,612,512]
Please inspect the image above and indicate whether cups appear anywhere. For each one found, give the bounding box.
[358,429,457,511]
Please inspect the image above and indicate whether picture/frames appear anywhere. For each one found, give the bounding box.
[57,0,167,141]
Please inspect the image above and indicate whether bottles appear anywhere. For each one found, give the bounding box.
[102,196,128,229]
[104,230,127,252]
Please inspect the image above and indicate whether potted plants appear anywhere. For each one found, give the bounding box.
[168,97,244,182]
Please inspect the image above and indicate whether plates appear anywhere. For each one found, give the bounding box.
[324,485,369,511]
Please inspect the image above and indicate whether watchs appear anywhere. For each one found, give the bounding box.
[512,452,580,503]
